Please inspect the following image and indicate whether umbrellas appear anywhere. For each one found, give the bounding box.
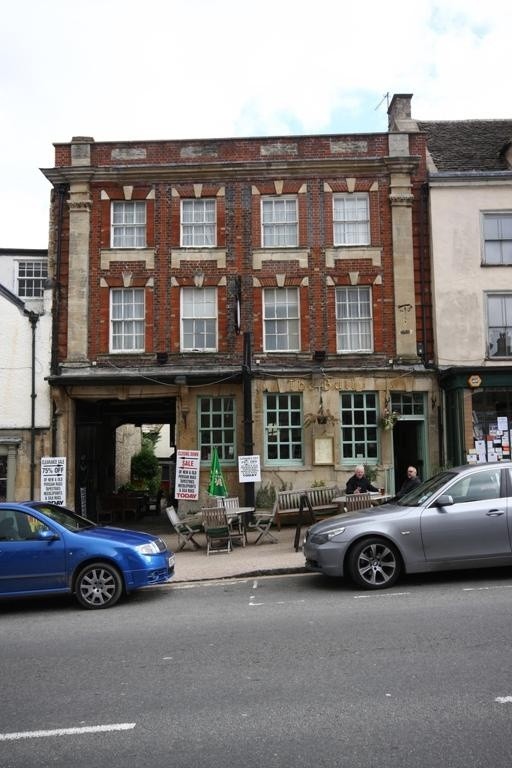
[204,445,229,507]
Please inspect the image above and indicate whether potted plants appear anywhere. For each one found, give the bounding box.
[301,407,343,437]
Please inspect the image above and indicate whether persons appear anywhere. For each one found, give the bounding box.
[382,464,422,504]
[345,464,381,506]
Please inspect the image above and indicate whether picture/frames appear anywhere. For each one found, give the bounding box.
[311,435,338,466]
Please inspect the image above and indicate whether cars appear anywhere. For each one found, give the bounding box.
[300,460,512,590]
[0,499,176,611]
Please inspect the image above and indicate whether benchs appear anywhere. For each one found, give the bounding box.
[274,483,344,532]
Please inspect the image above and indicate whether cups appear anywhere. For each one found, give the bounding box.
[381,489,385,495]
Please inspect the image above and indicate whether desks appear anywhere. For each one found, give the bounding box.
[195,506,254,548]
[119,496,146,522]
[331,492,397,514]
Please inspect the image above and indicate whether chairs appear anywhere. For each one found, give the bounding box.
[0,510,35,539]
[96,494,125,524]
[344,493,372,513]
[165,505,202,552]
[465,476,498,500]
[247,497,280,546]
[145,489,162,516]
[201,496,241,556]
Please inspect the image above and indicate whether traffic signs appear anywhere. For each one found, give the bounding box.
[173,449,202,501]
[39,456,68,508]
